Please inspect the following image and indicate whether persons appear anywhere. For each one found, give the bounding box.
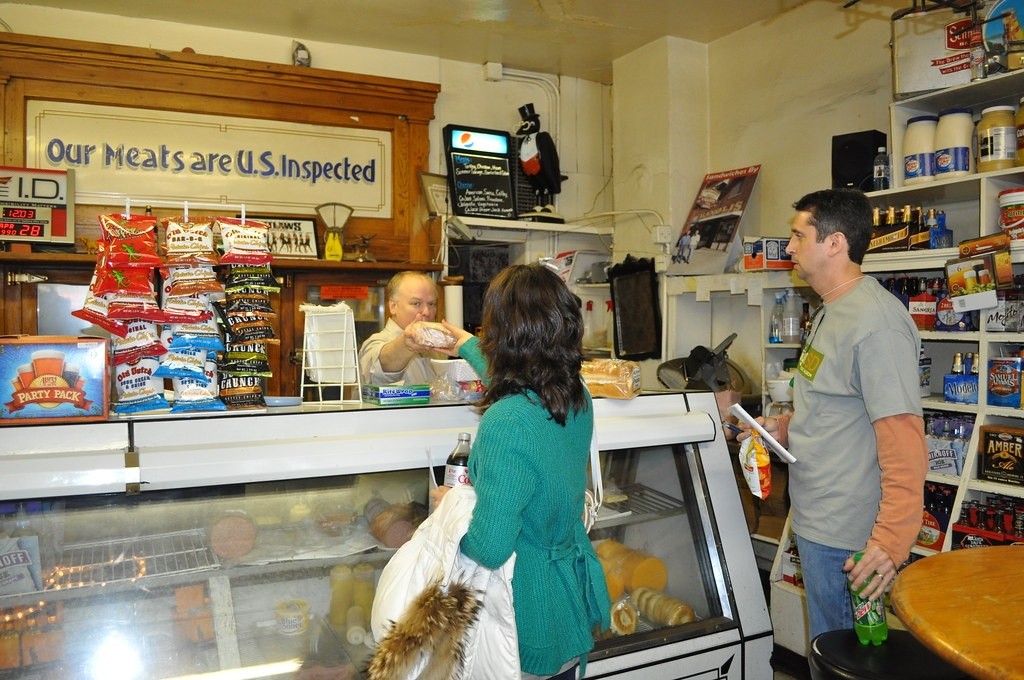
[686,230,700,263]
[673,230,691,264]
[737,188,929,639]
[350,271,439,516]
[266,232,313,253]
[431,263,610,680]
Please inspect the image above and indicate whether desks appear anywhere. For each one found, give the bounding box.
[891,546,1024,680]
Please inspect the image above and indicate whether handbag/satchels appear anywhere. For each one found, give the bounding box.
[573,373,603,535]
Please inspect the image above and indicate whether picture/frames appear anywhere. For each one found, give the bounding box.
[236,215,320,260]
[418,171,453,215]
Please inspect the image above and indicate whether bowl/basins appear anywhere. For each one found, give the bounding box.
[766,380,793,402]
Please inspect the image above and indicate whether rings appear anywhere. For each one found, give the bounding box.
[876,573,883,581]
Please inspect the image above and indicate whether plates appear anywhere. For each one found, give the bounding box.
[263,396,304,406]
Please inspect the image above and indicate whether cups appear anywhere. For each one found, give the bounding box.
[11,352,87,392]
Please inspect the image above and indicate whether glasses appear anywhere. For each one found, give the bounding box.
[800,305,826,354]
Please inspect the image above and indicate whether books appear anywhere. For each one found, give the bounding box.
[727,403,797,464]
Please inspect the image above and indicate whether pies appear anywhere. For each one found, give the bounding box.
[592,540,691,639]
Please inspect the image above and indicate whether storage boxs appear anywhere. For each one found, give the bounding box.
[979,426,1024,487]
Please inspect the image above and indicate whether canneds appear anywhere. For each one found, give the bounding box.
[970,46,987,81]
[960,497,1024,540]
[904,97,1024,184]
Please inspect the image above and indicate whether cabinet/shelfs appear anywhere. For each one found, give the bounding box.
[762,68,1024,656]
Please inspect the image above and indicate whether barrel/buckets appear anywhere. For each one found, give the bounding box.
[998,188,1024,241]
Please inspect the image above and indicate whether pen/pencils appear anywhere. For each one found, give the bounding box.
[723,423,745,434]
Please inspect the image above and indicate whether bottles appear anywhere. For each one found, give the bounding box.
[876,276,949,296]
[919,344,979,375]
[970,21,987,82]
[922,412,976,532]
[872,204,944,239]
[873,147,889,191]
[800,300,810,342]
[329,561,377,651]
[444,433,473,489]
[848,552,888,646]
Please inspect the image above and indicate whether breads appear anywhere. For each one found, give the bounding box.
[579,358,641,398]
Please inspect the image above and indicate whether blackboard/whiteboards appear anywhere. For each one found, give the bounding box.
[447,153,516,220]
